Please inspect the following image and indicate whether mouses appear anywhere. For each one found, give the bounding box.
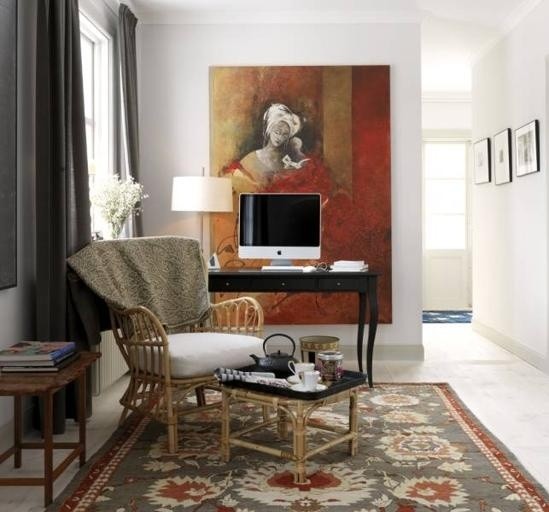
[303,266,317,273]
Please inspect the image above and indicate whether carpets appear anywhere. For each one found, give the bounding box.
[422,309,473,325]
[43,378,549,512]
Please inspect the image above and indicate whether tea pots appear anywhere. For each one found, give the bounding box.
[248,333,298,375]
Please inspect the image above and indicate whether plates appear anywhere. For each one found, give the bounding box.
[289,383,328,393]
[286,375,322,385]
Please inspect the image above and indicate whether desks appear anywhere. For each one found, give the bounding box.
[1,349,101,507]
[214,371,371,484]
[205,265,379,393]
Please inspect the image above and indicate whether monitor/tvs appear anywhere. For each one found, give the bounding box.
[237,193,322,271]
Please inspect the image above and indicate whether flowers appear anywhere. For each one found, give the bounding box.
[92,168,148,238]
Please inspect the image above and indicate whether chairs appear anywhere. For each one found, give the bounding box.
[90,238,270,451]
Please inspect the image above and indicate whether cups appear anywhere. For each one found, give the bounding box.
[300,335,340,363]
[288,359,315,379]
[299,372,320,389]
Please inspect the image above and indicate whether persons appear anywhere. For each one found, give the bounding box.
[232,102,304,190]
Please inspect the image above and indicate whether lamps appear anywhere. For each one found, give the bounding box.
[170,175,233,253]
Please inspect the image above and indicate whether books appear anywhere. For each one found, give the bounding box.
[0,337,81,373]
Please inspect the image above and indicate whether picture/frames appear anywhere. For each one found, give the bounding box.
[471,135,491,184]
[492,126,512,187]
[514,117,539,179]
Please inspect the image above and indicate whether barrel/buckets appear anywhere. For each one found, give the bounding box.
[299,335,340,372]
